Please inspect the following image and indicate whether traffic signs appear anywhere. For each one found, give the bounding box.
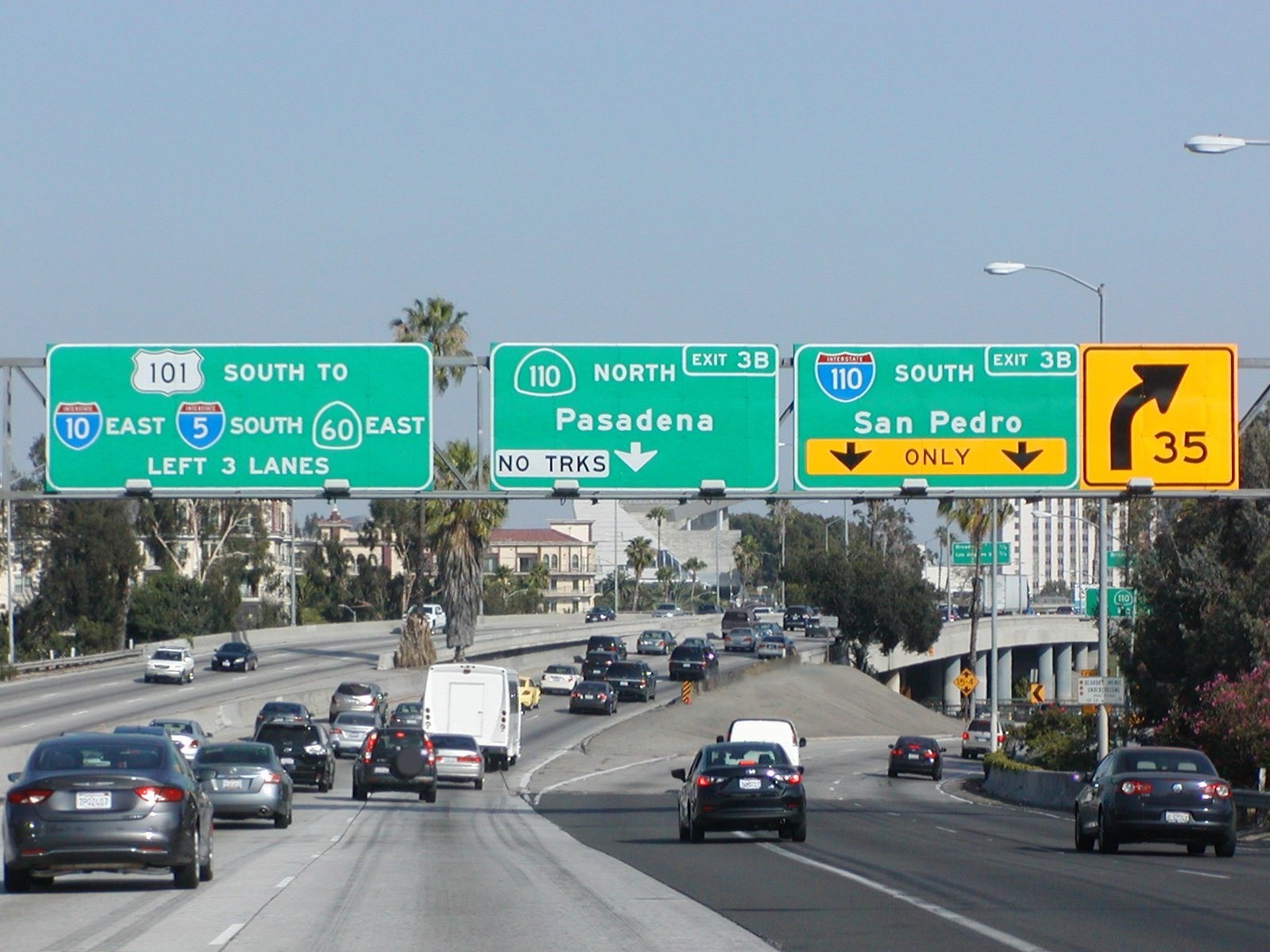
[487,340,782,502]
[791,341,1080,500]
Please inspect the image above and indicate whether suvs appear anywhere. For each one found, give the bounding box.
[143,645,195,685]
[351,726,439,804]
[402,604,447,635]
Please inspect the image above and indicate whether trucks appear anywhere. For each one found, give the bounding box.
[421,663,524,772]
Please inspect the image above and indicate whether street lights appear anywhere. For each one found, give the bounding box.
[982,261,1110,765]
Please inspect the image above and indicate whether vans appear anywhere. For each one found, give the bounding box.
[960,718,1016,760]
[715,718,808,767]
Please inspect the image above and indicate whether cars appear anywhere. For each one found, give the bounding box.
[535,605,963,716]
[191,740,295,829]
[887,735,948,781]
[327,681,390,724]
[670,741,808,843]
[327,711,382,761]
[1,732,220,894]
[584,606,616,624]
[112,701,339,792]
[211,641,259,673]
[651,602,684,618]
[1073,744,1239,858]
[425,733,488,792]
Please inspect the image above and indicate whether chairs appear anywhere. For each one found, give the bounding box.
[51,750,80,772]
[759,754,774,765]
[1178,762,1197,772]
[1136,760,1157,770]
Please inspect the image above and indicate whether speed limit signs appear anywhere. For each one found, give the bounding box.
[1078,339,1241,495]
